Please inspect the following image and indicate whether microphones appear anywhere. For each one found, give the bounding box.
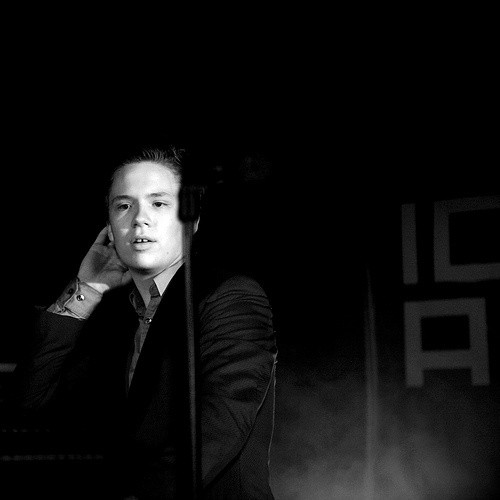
[180,154,203,221]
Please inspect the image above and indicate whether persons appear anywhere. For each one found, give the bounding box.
[15,140,276,500]
